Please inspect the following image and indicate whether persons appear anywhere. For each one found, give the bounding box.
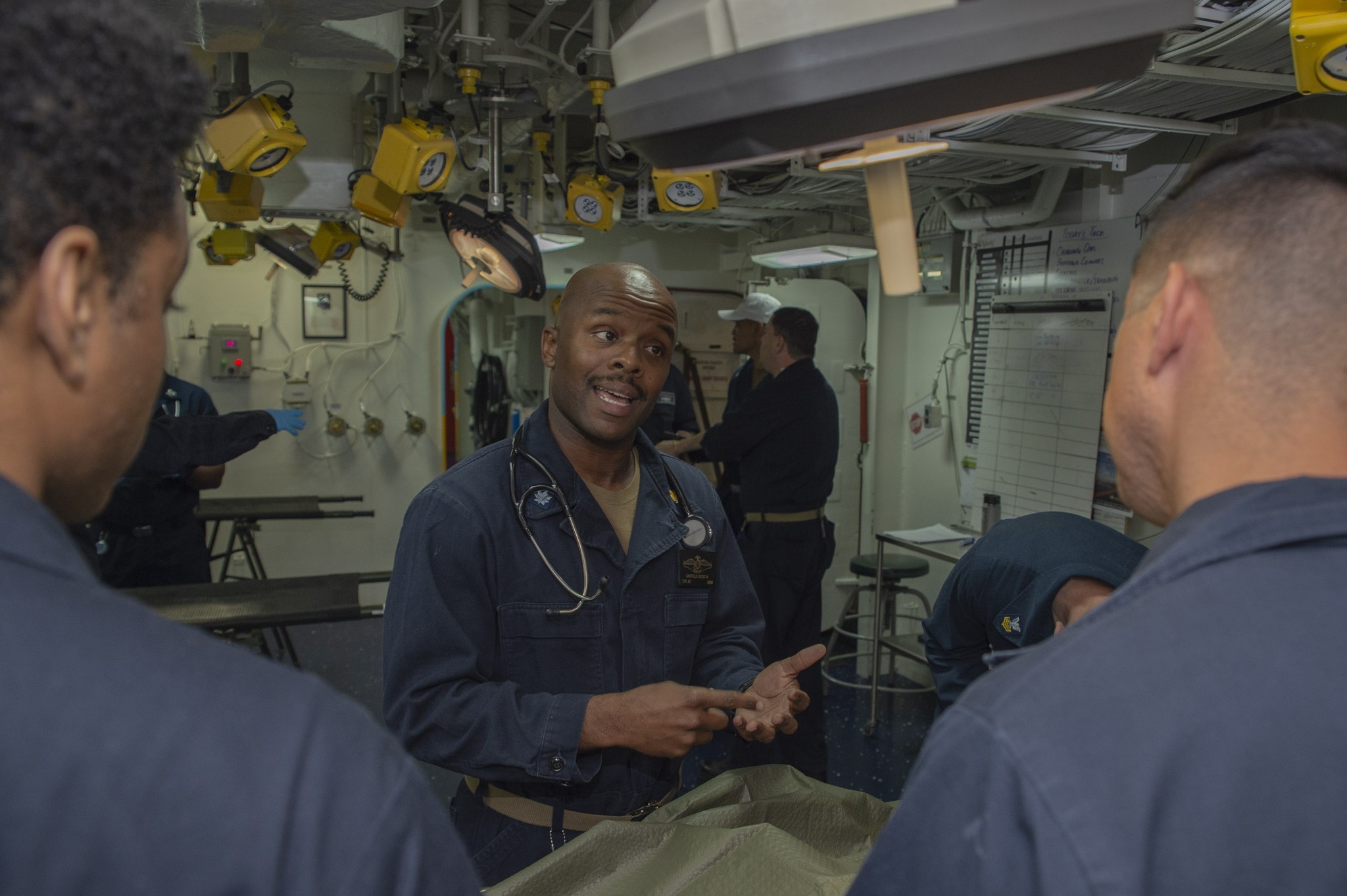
[388,262,826,886]
[0,0,483,896]
[920,511,1151,714]
[643,294,839,781]
[71,368,305,586]
[843,97,1347,896]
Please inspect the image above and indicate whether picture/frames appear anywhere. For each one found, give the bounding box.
[301,284,348,338]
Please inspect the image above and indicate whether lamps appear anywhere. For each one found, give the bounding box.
[749,233,877,270]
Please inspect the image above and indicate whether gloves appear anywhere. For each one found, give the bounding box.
[263,408,306,437]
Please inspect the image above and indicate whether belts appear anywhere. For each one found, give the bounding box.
[464,774,688,833]
[744,505,826,523]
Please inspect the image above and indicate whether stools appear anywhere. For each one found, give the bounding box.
[819,554,931,735]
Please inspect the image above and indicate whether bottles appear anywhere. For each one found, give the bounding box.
[981,493,1002,536]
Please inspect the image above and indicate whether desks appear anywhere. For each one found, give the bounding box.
[864,525,982,736]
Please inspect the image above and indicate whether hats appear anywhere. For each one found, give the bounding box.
[718,292,782,323]
